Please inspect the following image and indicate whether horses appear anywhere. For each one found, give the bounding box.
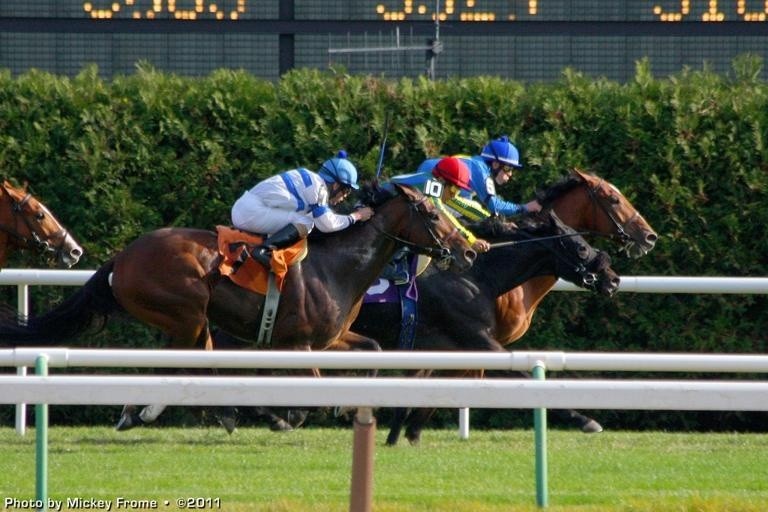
[0,178,480,437]
[0,178,86,270]
[207,206,621,436]
[384,167,660,447]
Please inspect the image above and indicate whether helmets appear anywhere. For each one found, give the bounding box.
[480,135,523,168]
[432,157,472,191]
[319,151,360,190]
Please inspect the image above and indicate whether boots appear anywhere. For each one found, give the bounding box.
[249,223,303,268]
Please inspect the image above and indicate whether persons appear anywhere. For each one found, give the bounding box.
[352,156,519,278]
[412,136,543,229]
[229,150,375,272]
